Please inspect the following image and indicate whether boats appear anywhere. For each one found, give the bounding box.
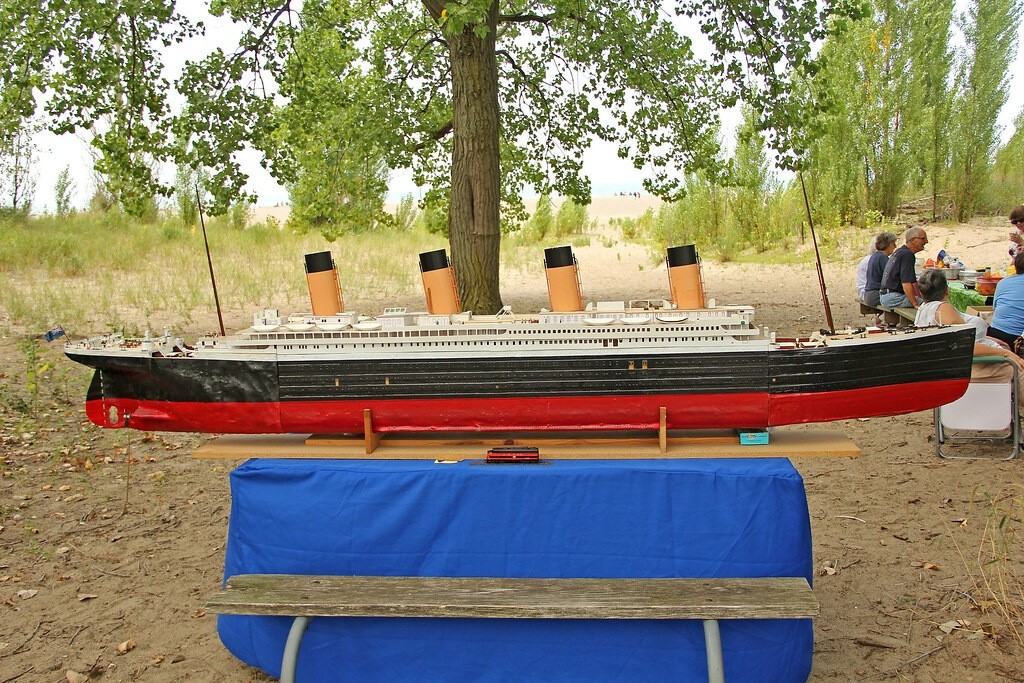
[64,171,977,434]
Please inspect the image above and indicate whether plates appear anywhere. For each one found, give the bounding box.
[317,324,346,330]
[287,325,315,331]
[251,325,277,331]
[656,317,688,323]
[583,319,613,325]
[619,318,651,324]
[353,324,382,330]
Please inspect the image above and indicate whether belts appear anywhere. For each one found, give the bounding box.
[880,289,904,295]
[864,288,880,292]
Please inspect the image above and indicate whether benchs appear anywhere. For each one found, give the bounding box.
[854,297,919,325]
[204,572,820,683]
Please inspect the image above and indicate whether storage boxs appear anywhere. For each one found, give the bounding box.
[966,306,995,320]
[974,277,1005,295]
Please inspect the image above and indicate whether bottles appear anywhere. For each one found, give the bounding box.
[984,267,992,282]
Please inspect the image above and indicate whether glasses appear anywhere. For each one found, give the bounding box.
[909,236,927,241]
[1011,217,1024,224]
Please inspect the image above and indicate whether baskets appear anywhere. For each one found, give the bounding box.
[976,276,1004,295]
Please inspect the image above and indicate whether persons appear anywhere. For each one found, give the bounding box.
[857,203,1024,357]
[915,267,1024,410]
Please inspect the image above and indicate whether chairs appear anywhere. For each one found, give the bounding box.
[933,358,1018,461]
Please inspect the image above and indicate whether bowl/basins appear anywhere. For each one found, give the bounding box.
[942,268,962,279]
